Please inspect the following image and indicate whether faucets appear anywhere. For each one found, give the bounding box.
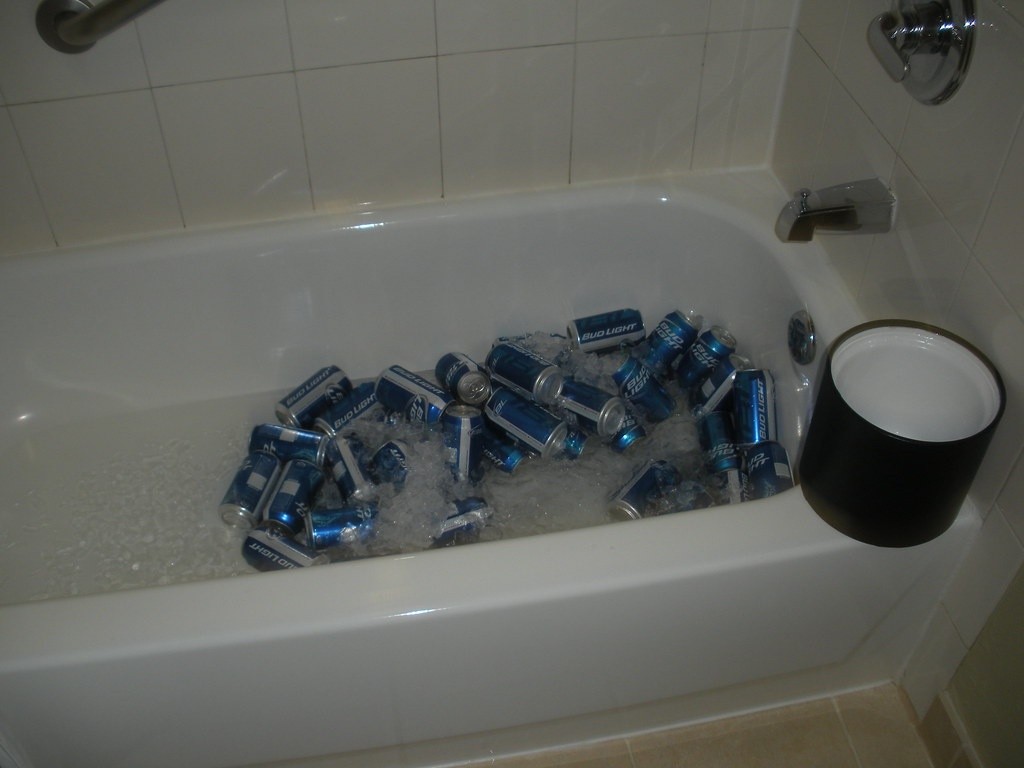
[774,177,898,245]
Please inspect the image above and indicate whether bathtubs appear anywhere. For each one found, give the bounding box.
[0,166,997,768]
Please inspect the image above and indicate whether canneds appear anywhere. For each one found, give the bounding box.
[219,308,794,572]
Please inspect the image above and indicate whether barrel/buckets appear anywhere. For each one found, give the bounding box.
[800,316,1006,547]
